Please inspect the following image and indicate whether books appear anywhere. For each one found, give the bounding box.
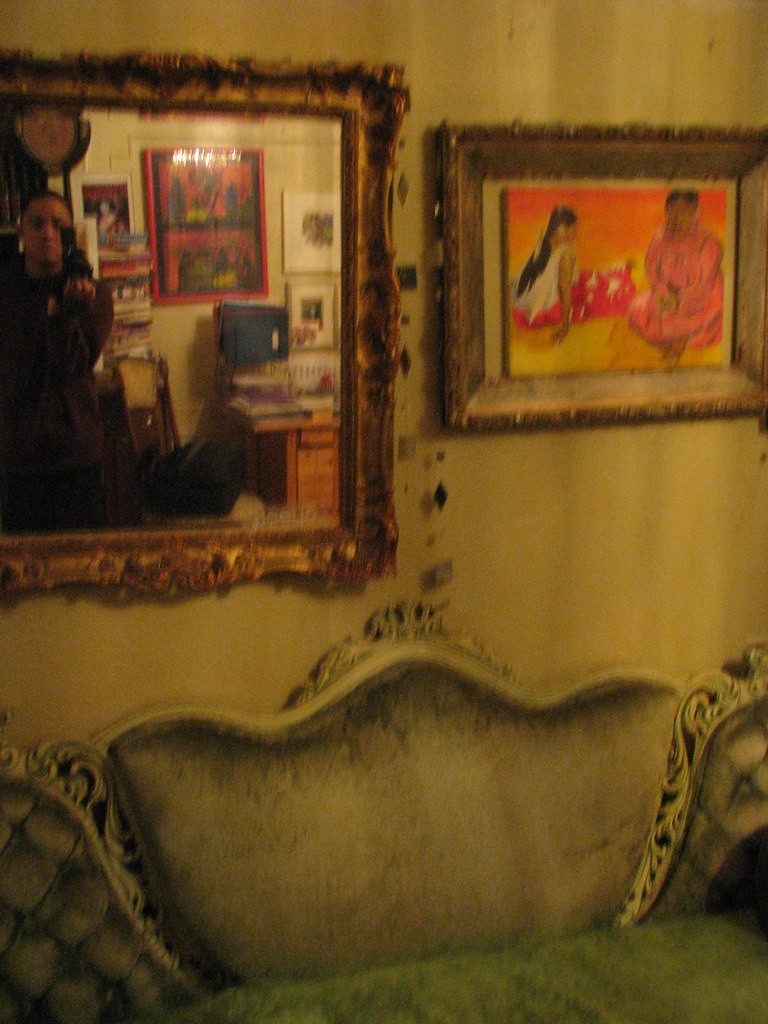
[96,228,154,366]
[233,390,301,419]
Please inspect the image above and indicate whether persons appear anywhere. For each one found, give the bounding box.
[0,191,115,533]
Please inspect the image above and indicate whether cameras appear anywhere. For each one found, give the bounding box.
[61,228,95,280]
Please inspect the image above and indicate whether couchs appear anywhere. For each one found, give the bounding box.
[0,600,768,1024]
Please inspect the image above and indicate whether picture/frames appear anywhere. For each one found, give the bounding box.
[0,46,413,600]
[431,120,768,431]
